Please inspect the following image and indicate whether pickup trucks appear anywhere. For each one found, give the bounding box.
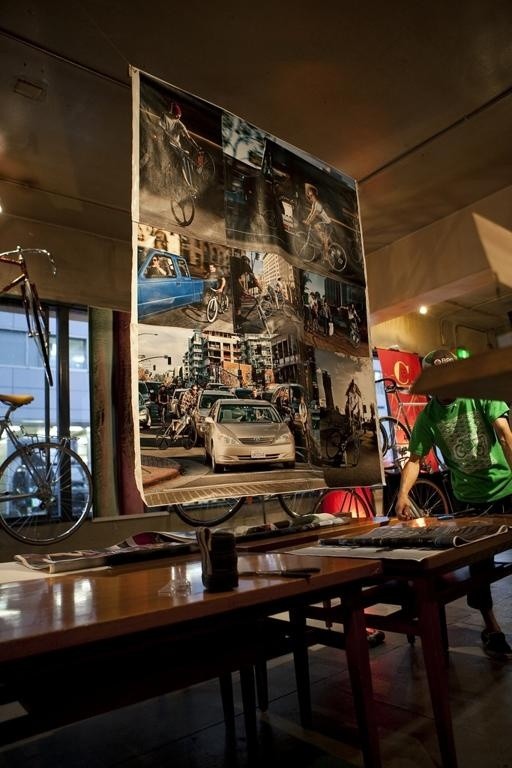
[137,247,202,320]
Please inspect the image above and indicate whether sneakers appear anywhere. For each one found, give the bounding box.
[481,629,512,660]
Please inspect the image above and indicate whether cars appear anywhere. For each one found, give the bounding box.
[139,381,308,473]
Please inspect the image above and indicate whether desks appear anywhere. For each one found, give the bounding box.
[0,517,512,764]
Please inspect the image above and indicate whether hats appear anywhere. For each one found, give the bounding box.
[422,349,457,371]
[170,102,181,115]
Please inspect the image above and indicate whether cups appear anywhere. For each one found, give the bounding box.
[195,527,238,590]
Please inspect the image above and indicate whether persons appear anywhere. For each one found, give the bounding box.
[203,263,228,308]
[393,350,512,655]
[228,253,361,342]
[301,183,333,261]
[150,384,198,436]
[256,389,312,464]
[344,384,362,438]
[147,255,166,277]
[144,105,203,196]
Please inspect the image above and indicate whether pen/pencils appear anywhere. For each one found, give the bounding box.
[256,568,321,578]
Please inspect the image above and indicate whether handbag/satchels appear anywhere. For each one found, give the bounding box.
[328,322,335,336]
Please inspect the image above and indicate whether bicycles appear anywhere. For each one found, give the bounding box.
[325,422,360,467]
[292,221,347,273]
[1,245,95,546]
[204,285,361,348]
[175,490,324,528]
[314,377,449,519]
[171,151,216,226]
